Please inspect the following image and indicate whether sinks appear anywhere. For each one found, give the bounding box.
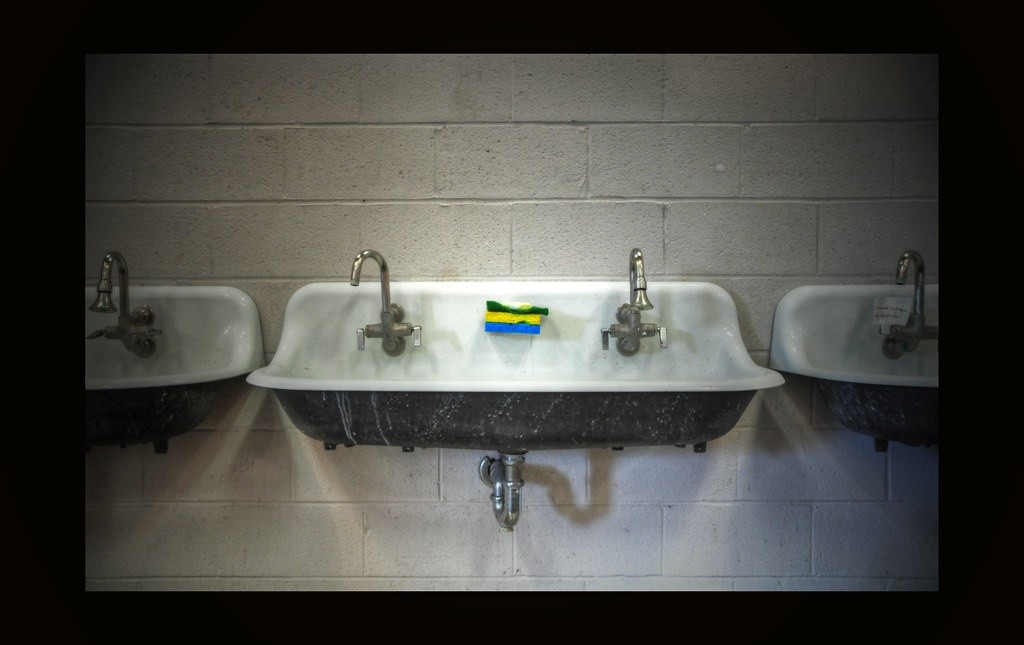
[85,282,265,447]
[769,281,938,443]
[242,279,789,452]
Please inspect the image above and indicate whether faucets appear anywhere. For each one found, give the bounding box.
[879,249,937,361]
[600,246,670,355]
[88,250,164,358]
[345,246,422,355]
